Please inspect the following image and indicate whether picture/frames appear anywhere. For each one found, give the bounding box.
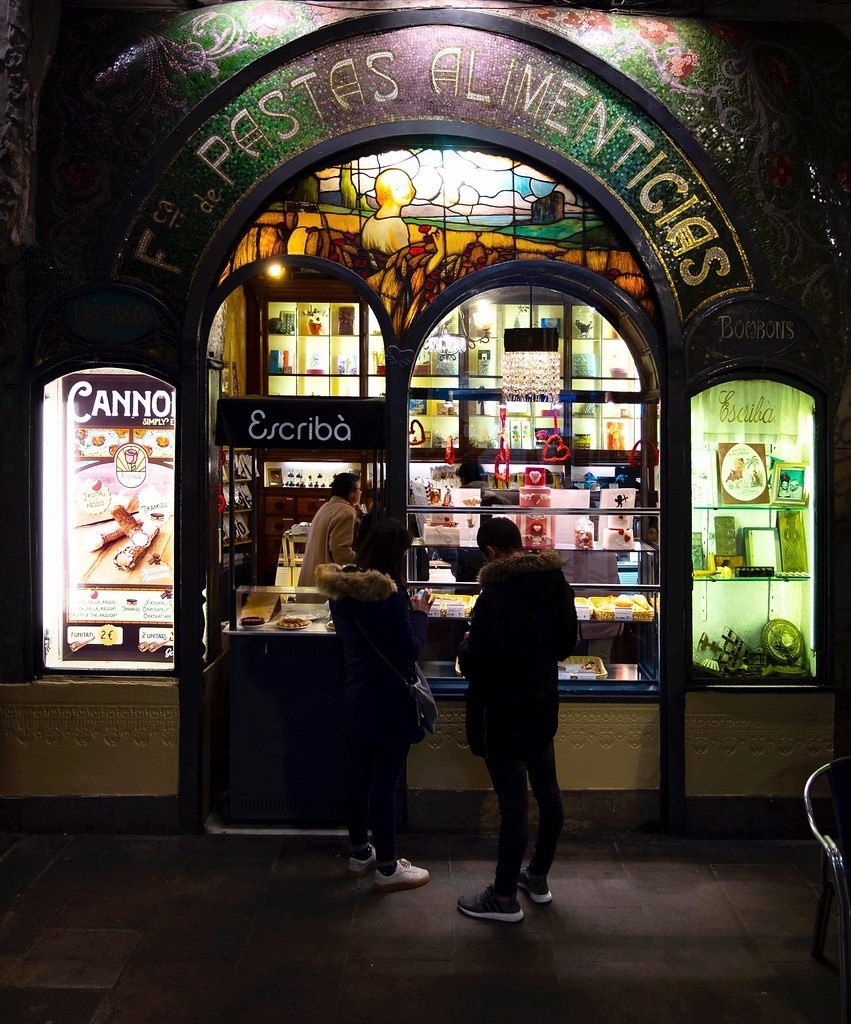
[770,463,810,506]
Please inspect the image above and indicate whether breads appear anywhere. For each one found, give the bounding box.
[599,594,652,611]
[240,616,265,625]
[85,505,159,571]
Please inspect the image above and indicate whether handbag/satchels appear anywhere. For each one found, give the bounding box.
[396,662,437,735]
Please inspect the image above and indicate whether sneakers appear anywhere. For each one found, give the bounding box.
[375,856,430,892]
[348,843,377,878]
[518,865,553,903]
[458,882,524,923]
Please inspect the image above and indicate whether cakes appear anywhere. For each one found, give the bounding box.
[602,513,634,551]
[515,466,555,548]
[423,480,460,547]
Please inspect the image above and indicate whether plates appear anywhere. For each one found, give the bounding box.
[238,623,266,630]
[326,620,335,630]
[290,615,319,620]
[274,621,312,629]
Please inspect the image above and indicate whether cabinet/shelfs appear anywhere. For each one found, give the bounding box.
[693,506,811,582]
[218,302,660,687]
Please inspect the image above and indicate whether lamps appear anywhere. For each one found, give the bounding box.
[502,286,560,405]
[422,304,493,362]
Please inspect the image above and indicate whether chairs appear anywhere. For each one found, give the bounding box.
[803,755,851,1024]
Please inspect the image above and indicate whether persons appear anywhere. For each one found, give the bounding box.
[455,462,514,583]
[334,518,432,889]
[296,473,361,604]
[457,516,579,921]
[561,485,624,665]
[778,472,802,499]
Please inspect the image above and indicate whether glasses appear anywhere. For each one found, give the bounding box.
[356,486,363,493]
